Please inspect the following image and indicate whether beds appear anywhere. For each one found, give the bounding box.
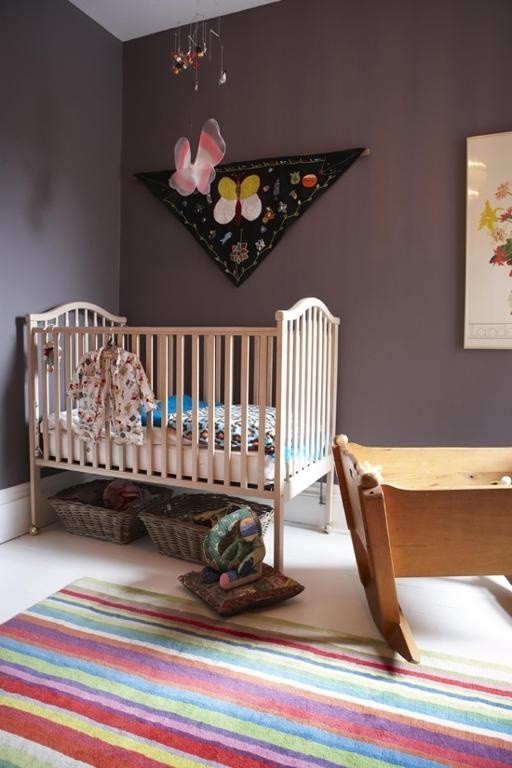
[328,433,512,663]
[26,301,341,574]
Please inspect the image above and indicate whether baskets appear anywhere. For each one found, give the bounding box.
[47,479,173,544]
[137,494,275,567]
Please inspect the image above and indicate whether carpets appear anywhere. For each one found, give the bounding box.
[1,575,512,768]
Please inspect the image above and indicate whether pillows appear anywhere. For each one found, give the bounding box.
[168,404,298,458]
[177,562,305,618]
